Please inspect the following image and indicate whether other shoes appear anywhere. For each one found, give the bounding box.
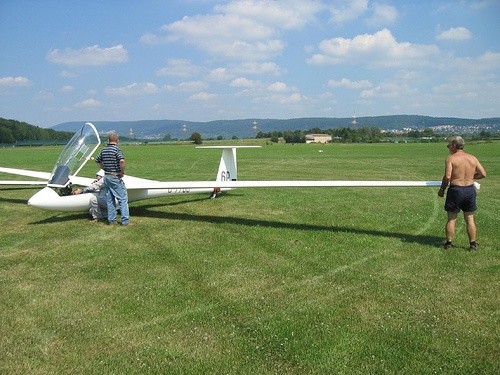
[444,245,451,249]
[470,243,479,252]
[123,221,134,226]
[90,218,102,223]
[109,221,122,225]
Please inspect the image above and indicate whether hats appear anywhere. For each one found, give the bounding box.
[96,169,105,176]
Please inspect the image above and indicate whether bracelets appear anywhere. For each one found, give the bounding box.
[441,187,446,191]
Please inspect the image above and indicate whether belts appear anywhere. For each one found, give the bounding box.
[105,173,119,176]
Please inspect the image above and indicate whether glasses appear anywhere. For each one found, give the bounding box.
[447,144,453,148]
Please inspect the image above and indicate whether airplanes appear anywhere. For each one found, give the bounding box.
[0,125,479,231]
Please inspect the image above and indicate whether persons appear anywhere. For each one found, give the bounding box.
[438,135,486,252]
[94,132,134,226]
[71,168,108,222]
